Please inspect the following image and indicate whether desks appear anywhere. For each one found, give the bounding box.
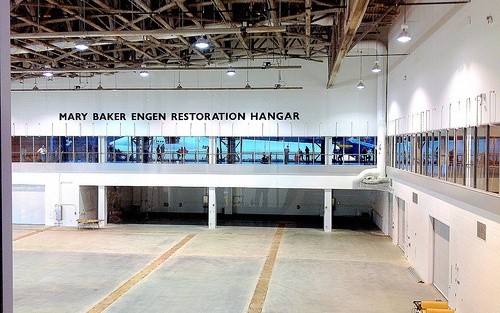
[413,300,451,313]
[75,219,103,230]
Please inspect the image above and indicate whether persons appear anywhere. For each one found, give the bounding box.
[284,146,454,166]
[20,144,220,164]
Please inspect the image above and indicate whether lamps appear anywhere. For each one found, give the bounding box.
[397,7,412,43]
[226,64,236,75]
[244,58,252,89]
[139,42,150,76]
[357,51,365,89]
[371,32,382,73]
[43,47,53,77]
[75,0,89,49]
[195,0,209,50]
[271,46,276,66]
